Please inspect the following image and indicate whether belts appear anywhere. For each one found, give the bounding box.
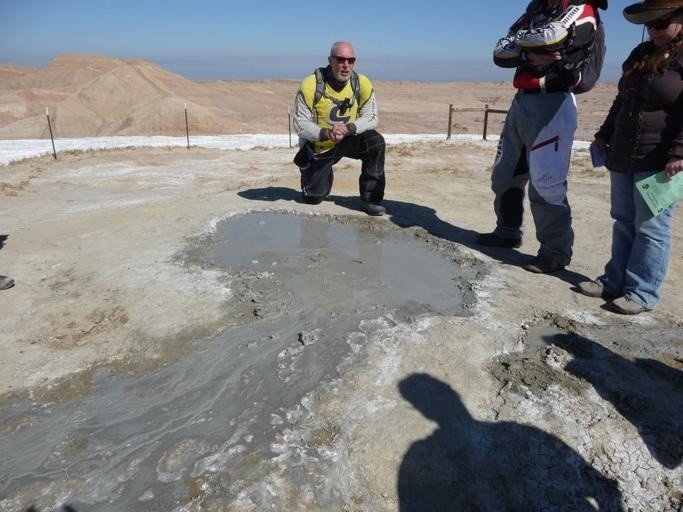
[519,88,568,94]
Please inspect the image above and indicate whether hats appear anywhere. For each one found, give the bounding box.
[623,0,683,24]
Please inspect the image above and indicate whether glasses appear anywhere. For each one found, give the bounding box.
[330,56,355,64]
[644,12,679,30]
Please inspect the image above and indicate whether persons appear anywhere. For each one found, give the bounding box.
[290,39,387,216]
[474,1,609,274]
[576,1,683,316]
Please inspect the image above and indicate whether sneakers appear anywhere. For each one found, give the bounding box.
[475,233,521,247]
[528,256,564,272]
[611,295,647,314]
[360,201,386,215]
[578,281,618,297]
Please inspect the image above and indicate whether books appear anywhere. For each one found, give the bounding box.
[634,170,683,217]
[588,143,608,167]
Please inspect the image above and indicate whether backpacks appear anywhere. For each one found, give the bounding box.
[572,12,605,94]
